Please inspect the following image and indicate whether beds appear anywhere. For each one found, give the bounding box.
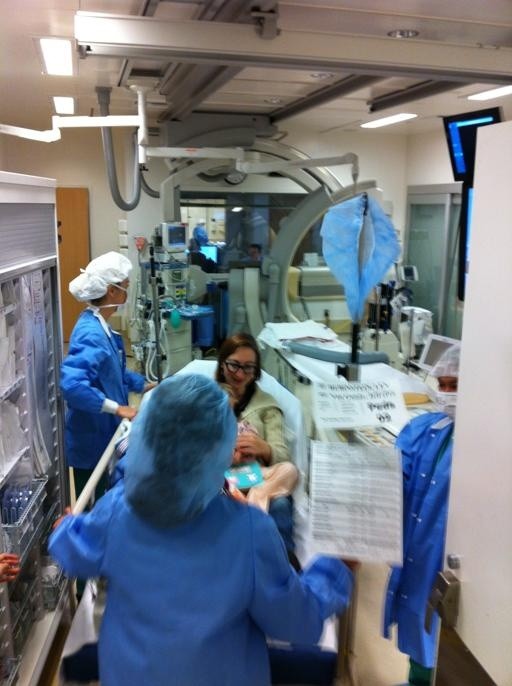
[69,361,339,685]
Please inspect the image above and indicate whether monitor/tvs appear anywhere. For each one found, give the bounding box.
[162,223,189,253]
[457,182,474,301]
[442,107,503,181]
[186,246,218,265]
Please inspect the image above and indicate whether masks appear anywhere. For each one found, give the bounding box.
[115,284,130,312]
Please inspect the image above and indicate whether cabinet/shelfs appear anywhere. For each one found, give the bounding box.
[0,173,77,686]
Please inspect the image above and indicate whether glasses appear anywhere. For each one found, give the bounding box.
[223,361,256,374]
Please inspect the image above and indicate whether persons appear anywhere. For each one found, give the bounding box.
[238,243,262,268]
[187,237,206,267]
[46,372,366,684]
[219,382,299,515]
[0,554,23,584]
[378,342,462,684]
[193,217,209,246]
[214,333,300,516]
[58,248,160,607]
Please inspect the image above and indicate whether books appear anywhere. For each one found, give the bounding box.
[225,462,262,490]
